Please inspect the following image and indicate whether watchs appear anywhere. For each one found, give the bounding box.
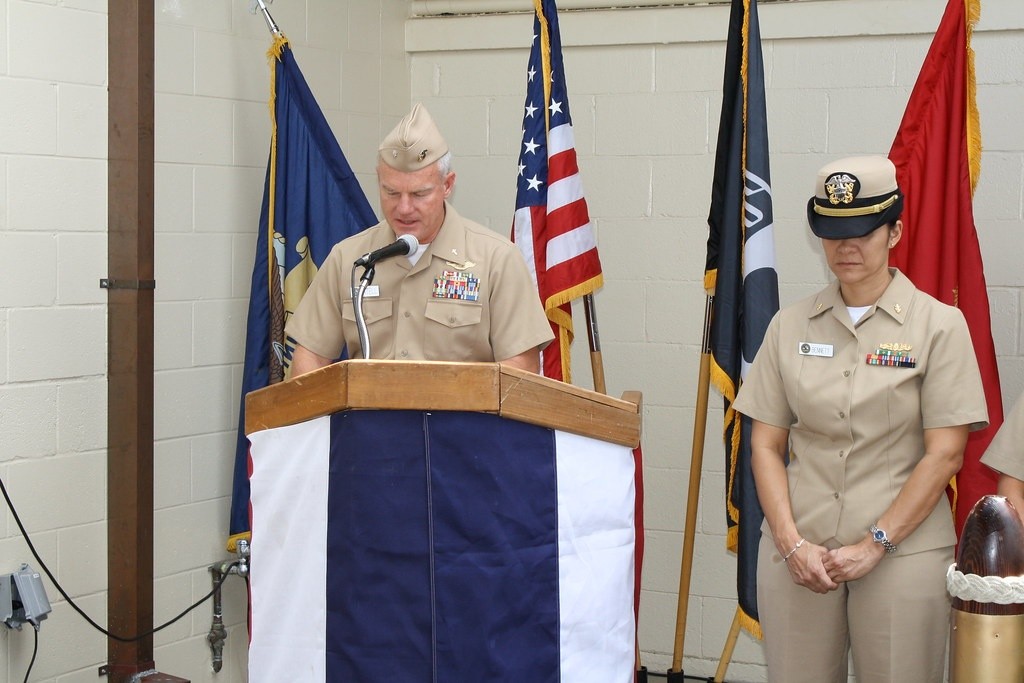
[869,524,896,555]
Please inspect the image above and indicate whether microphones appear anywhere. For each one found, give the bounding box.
[354,234,420,267]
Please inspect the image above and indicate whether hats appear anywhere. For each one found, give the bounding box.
[806,155,903,239]
[378,101,448,171]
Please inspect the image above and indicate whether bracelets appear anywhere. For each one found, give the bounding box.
[783,538,806,562]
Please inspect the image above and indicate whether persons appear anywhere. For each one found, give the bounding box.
[978,391,1024,530]
[729,155,992,683]
[283,101,556,383]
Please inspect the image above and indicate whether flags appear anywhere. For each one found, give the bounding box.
[704,0,797,643]
[226,37,386,554]
[874,0,1006,566]
[511,1,600,386]
